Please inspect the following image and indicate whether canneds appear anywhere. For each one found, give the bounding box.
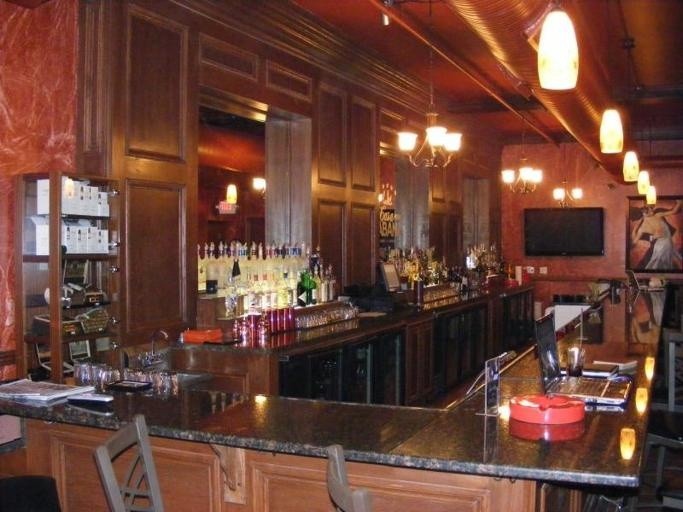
[233,306,294,346]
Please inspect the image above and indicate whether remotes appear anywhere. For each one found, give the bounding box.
[67,394,115,406]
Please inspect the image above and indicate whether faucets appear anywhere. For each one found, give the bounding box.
[151,329,168,357]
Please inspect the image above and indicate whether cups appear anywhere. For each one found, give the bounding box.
[74,362,178,390]
[515,265,523,285]
[568,348,585,376]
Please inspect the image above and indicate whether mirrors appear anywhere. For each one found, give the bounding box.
[198,103,265,260]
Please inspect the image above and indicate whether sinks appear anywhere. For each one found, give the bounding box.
[176,367,213,389]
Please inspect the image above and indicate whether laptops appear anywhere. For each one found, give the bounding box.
[533,312,633,408]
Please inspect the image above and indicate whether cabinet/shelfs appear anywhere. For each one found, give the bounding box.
[18,174,121,384]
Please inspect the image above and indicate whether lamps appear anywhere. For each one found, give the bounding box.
[225,183,238,205]
[597,108,624,154]
[549,176,587,207]
[645,185,656,204]
[622,150,639,183]
[394,102,463,168]
[536,8,580,92]
[499,151,546,195]
[636,170,650,195]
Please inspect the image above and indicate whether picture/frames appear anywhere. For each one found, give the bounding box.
[624,195,682,273]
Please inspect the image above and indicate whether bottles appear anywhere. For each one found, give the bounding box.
[198,240,336,348]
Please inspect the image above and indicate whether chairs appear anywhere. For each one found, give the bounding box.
[327,444,369,512]
[93,415,164,512]
[581,284,683,512]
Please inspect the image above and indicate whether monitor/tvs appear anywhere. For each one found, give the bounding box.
[524,207,605,259]
